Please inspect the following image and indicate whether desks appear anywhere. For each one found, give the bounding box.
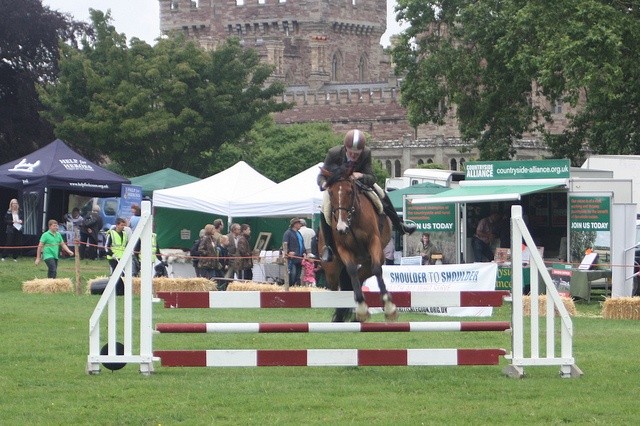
[568,267,611,304]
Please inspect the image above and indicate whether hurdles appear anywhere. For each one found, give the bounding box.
[89,201,582,377]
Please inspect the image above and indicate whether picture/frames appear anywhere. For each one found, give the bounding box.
[578,252,598,270]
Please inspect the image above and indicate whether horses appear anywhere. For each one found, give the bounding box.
[317,163,400,323]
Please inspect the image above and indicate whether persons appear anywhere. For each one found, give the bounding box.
[197,224,217,281]
[35,219,74,278]
[152,225,165,280]
[63,207,83,230]
[226,222,242,282]
[317,128,415,261]
[1,198,24,263]
[214,219,225,232]
[299,219,317,286]
[415,232,443,265]
[190,228,206,277]
[282,217,307,287]
[559,229,567,263]
[79,204,103,259]
[382,236,395,265]
[104,218,139,277]
[237,224,253,280]
[523,238,530,262]
[216,234,229,287]
[129,203,141,231]
[300,252,322,287]
[472,212,503,262]
[122,219,141,272]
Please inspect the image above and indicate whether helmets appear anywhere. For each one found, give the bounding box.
[344,129,364,154]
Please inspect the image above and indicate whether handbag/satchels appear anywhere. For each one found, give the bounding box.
[85,217,96,227]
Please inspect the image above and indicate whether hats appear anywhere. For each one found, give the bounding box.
[307,253,315,258]
[289,216,300,228]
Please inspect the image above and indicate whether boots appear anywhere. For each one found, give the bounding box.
[380,189,417,234]
[320,211,333,261]
